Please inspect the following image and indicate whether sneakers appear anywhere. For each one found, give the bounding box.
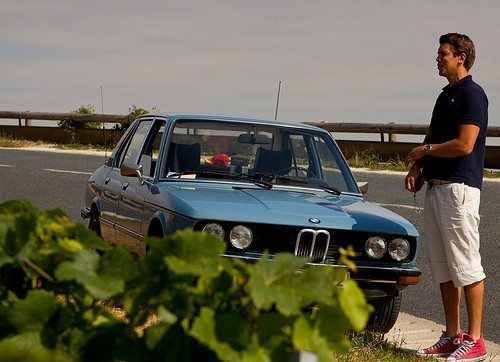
[446,334,487,362]
[416,330,463,356]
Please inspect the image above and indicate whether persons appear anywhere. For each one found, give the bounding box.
[405,33,489,362]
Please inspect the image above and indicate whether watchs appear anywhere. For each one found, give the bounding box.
[425,144,432,153]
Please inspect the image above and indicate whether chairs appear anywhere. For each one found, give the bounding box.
[163,142,201,174]
[254,147,293,177]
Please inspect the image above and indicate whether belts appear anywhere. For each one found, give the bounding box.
[430,179,451,184]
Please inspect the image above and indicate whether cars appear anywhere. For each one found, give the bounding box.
[79,112,422,334]
